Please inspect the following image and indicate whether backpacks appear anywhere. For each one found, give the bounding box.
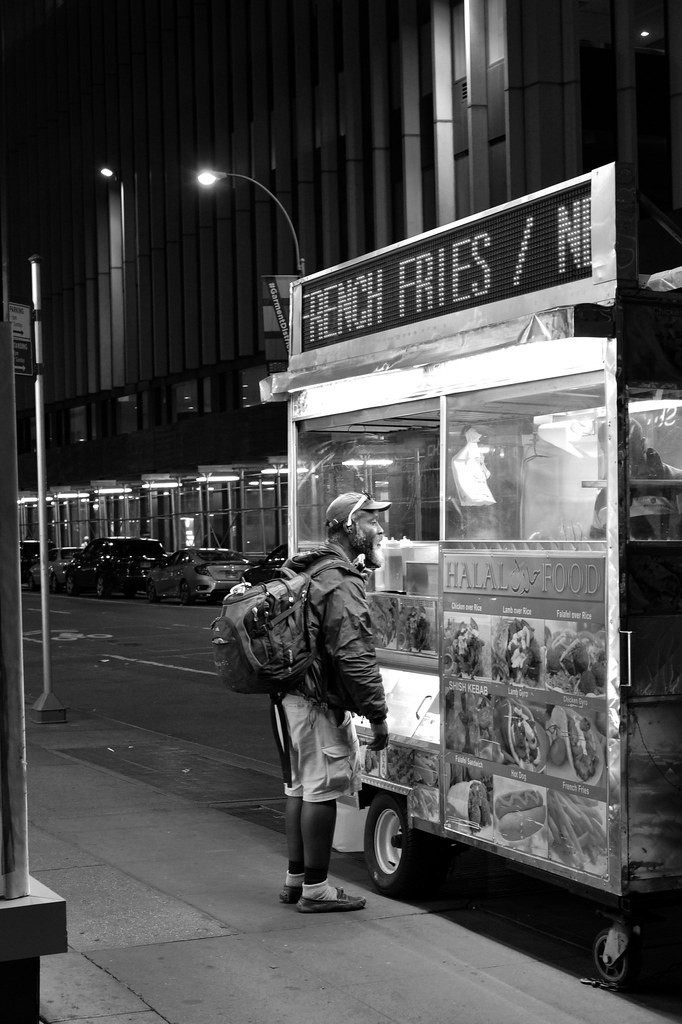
[210,548,365,789]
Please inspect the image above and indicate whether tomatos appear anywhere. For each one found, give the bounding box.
[550,737,566,765]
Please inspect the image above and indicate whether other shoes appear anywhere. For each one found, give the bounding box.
[296,887,366,913]
[279,882,302,903]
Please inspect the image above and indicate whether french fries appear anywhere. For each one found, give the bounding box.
[548,788,606,868]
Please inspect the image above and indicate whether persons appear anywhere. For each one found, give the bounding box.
[589,418,682,541]
[275,492,389,913]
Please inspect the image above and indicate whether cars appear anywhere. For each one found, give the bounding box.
[28,546,84,591]
[240,542,326,587]
[146,548,252,604]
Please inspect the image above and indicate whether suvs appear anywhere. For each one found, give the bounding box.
[63,536,168,600]
[20,540,57,584]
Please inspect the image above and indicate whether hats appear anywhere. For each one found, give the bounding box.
[325,492,392,528]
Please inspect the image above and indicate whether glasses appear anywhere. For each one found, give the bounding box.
[346,492,371,526]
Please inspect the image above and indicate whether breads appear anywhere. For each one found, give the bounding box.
[495,790,546,841]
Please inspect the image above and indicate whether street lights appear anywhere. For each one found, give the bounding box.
[198,170,305,279]
